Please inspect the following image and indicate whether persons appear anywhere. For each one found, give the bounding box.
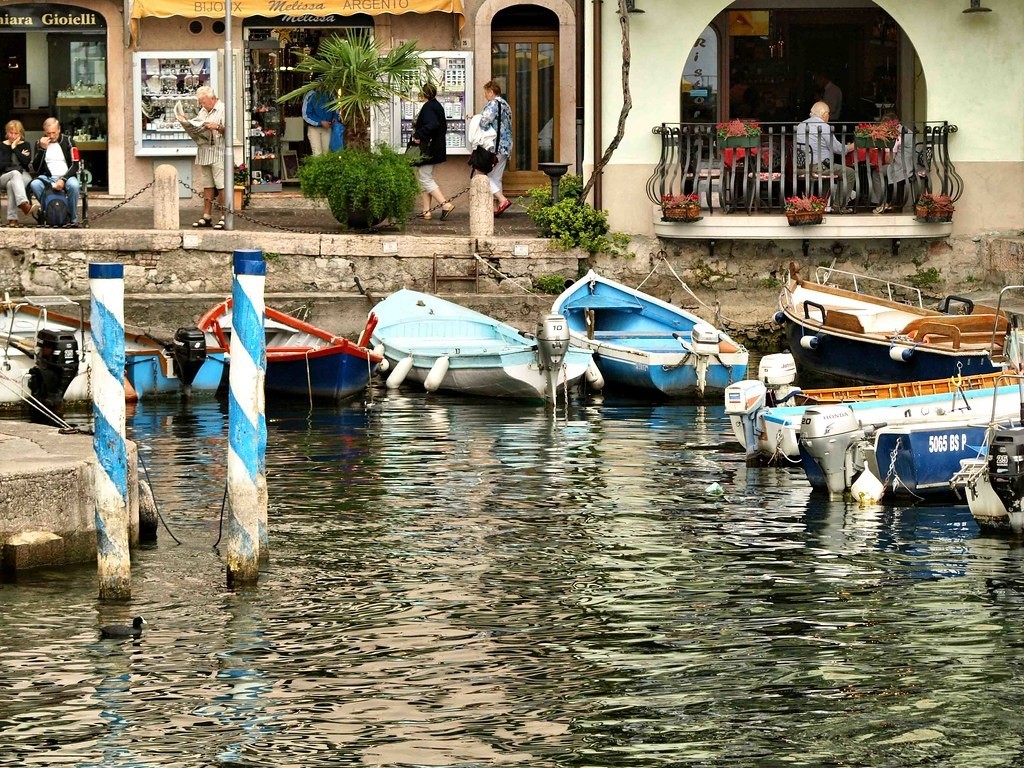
[413,83,455,221]
[145,76,162,94]
[466,81,514,217]
[797,101,855,213]
[0,120,39,226]
[813,71,842,130]
[189,58,204,75]
[183,86,226,229]
[30,117,78,227]
[302,72,340,158]
[871,112,922,214]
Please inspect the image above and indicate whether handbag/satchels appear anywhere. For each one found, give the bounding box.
[329,121,346,153]
[406,140,434,166]
[468,145,499,179]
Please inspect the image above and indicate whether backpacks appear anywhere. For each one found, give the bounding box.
[25,177,71,227]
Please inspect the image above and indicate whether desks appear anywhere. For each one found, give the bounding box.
[847,149,895,212]
[719,147,775,213]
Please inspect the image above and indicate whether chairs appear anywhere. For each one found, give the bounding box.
[789,142,844,215]
[678,143,719,215]
[750,141,789,211]
[900,141,933,212]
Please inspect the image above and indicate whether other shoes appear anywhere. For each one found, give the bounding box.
[831,206,853,214]
[872,203,892,214]
[494,200,511,216]
[21,202,35,217]
[10,220,17,226]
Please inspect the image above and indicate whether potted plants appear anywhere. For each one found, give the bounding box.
[271,25,442,242]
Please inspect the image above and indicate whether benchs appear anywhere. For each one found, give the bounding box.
[0,159,91,228]
[640,347,687,352]
[583,330,691,339]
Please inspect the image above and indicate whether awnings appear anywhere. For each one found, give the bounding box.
[123,0,467,51]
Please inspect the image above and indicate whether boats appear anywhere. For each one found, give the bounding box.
[723,345,1024,548]
[360,285,595,412]
[195,296,384,408]
[0,299,227,427]
[550,267,750,404]
[774,260,1024,389]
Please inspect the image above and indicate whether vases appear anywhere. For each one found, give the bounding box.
[786,210,823,227]
[716,136,761,148]
[916,206,954,223]
[663,207,699,219]
[855,135,896,149]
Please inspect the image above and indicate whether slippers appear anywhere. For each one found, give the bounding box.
[439,204,455,221]
[416,212,431,220]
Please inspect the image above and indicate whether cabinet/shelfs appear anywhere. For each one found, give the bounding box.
[248,41,283,194]
[56,97,106,152]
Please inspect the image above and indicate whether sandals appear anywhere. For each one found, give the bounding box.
[193,217,212,227]
[213,219,226,230]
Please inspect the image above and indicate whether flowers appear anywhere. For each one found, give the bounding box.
[716,117,760,138]
[917,192,956,212]
[661,193,701,208]
[854,122,901,146]
[784,192,830,211]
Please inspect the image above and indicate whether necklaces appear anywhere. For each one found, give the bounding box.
[142,100,154,119]
[217,124,220,129]
[191,59,202,65]
[427,69,444,87]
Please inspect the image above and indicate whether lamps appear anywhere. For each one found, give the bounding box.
[962,0,992,13]
